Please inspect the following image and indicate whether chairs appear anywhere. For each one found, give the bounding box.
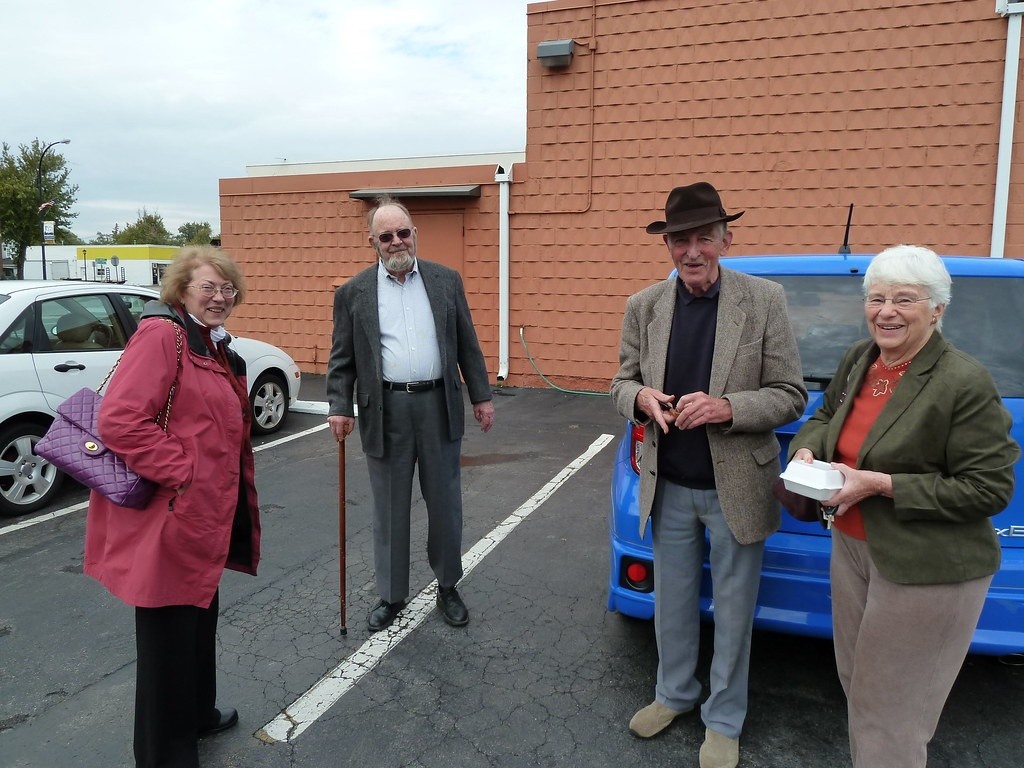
[1,317,24,349]
[54,314,105,351]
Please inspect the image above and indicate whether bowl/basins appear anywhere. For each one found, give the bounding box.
[779,459,846,501]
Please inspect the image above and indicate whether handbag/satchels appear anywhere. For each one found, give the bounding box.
[34,386,159,509]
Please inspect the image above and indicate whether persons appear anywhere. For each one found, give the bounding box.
[787,245,1021,768]
[85,243,260,768]
[325,205,494,630]
[610,181,810,768]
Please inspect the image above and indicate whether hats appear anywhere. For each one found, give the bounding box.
[645,182,746,236]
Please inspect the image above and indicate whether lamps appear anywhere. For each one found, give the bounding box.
[537,39,575,66]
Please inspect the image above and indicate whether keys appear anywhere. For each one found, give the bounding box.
[821,504,839,533]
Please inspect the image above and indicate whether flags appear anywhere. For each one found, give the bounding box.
[37,200,55,214]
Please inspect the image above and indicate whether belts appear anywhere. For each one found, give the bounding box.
[383,376,443,393]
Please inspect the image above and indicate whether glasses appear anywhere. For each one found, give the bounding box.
[183,284,239,299]
[863,293,933,311]
[373,229,413,243]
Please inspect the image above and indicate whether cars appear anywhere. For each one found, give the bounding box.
[0,279,300,521]
[606,256,1024,658]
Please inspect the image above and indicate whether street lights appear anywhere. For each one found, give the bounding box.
[82,249,88,282]
[37,139,72,280]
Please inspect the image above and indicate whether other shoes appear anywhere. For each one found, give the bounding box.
[699,728,740,767]
[628,703,694,739]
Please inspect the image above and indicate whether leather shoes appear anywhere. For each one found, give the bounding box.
[367,598,406,631]
[436,589,469,626]
[205,707,238,734]
